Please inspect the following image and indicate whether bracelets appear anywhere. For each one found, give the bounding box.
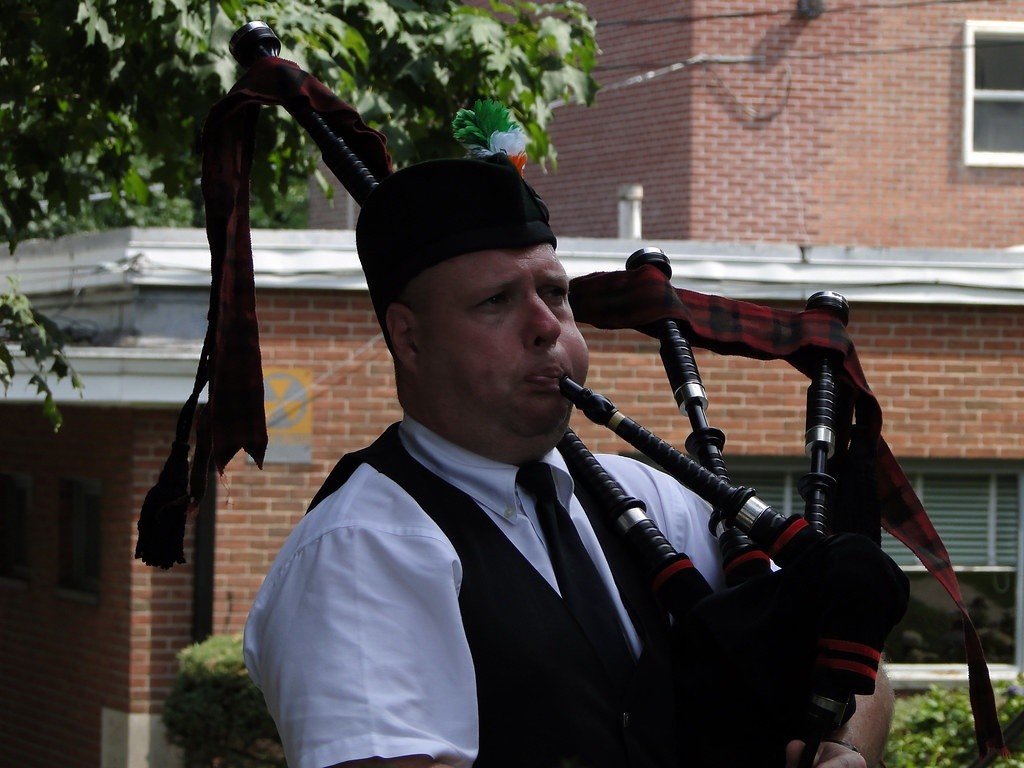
[829,738,859,753]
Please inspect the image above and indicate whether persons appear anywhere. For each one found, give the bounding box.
[244,155,895,768]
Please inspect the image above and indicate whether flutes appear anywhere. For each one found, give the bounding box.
[226,21,908,768]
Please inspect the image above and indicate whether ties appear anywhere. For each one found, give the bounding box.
[517,460,636,666]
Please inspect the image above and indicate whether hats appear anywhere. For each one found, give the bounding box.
[356,152,557,308]
